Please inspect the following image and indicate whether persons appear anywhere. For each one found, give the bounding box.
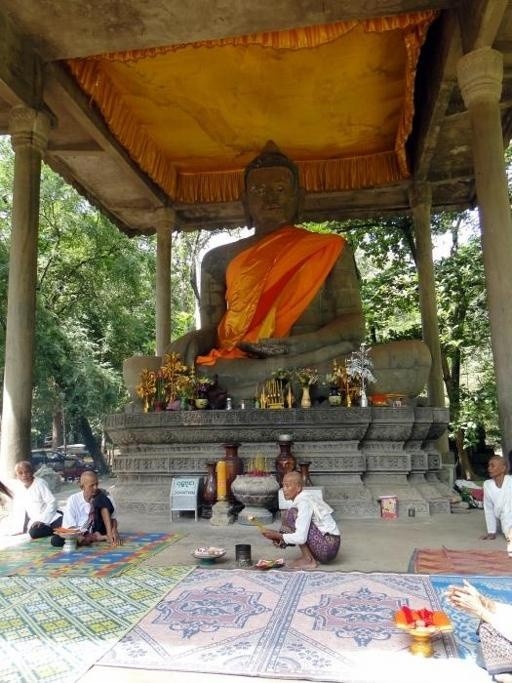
[122,140,433,399]
[258,472,341,568]
[51,470,124,549]
[445,577,512,683]
[8,461,63,539]
[479,456,512,557]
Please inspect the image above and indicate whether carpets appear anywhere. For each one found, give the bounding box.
[0,531,512,682]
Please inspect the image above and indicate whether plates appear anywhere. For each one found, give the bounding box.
[191,547,227,561]
[52,529,85,539]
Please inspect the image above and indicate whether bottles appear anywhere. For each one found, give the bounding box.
[226,398,233,410]
[239,400,246,409]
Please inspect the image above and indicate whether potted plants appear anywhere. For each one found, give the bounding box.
[189,375,215,409]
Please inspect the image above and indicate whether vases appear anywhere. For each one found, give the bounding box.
[290,367,319,408]
[358,388,368,406]
[142,395,150,413]
[344,383,352,406]
[179,396,188,410]
[152,400,164,411]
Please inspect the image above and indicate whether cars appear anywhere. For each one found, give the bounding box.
[31,436,120,482]
